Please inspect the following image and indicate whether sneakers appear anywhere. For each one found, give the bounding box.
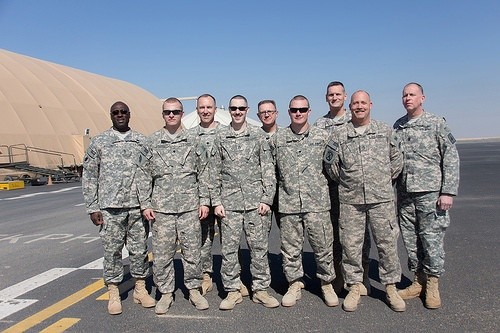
[219,291,242,309]
[253,289,279,307]
[155,292,174,314]
[189,289,209,309]
[299,279,307,289]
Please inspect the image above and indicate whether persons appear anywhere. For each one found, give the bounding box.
[81,102,156,315]
[208,94,280,310]
[322,88,408,312]
[187,80,371,309]
[269,95,340,308]
[133,97,210,314]
[392,82,460,309]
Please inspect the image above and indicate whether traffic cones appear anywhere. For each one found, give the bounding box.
[47,176,53,185]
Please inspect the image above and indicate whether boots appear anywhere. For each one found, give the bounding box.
[239,278,252,296]
[281,279,302,306]
[426,275,441,308]
[398,269,425,300]
[343,283,360,311]
[133,278,155,307]
[320,278,338,307]
[332,268,343,295]
[359,263,372,295]
[200,272,213,296]
[107,282,122,314]
[386,284,406,312]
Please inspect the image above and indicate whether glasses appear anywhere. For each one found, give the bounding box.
[229,107,247,111]
[259,110,277,114]
[112,110,127,115]
[290,108,308,113]
[163,110,184,115]
